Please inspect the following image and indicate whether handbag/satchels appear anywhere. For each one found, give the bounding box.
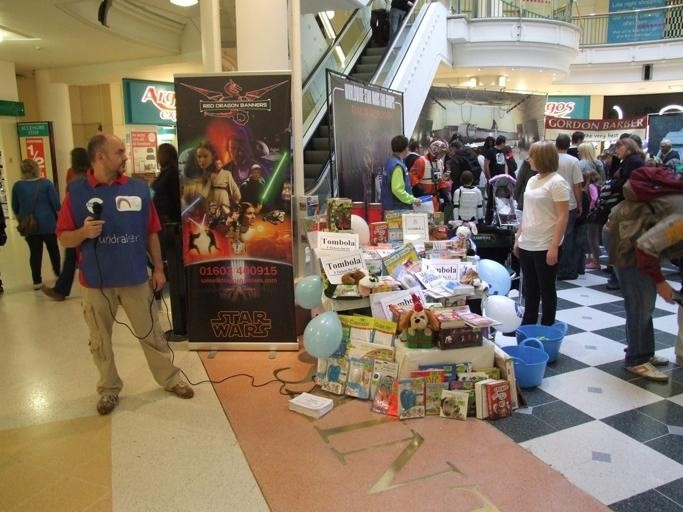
[17,213,39,238]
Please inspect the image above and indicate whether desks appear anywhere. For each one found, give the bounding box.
[320,280,487,317]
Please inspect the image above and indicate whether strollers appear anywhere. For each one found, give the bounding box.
[489,174,520,231]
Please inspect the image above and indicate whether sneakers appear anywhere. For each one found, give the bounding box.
[96,394,120,416]
[647,355,669,366]
[41,283,66,302]
[584,258,601,269]
[163,379,194,399]
[605,280,620,291]
[33,282,46,290]
[624,361,669,382]
[163,329,187,342]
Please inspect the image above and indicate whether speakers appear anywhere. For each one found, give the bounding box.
[644,66,650,79]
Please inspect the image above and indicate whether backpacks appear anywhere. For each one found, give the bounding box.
[622,165,683,203]
[455,153,483,187]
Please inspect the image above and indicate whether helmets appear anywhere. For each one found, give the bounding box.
[428,140,448,160]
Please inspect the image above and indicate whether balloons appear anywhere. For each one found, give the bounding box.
[303,311,343,358]
[294,274,323,310]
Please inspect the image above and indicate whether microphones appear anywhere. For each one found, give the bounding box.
[92,202,103,245]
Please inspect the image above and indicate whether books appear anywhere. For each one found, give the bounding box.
[307,215,519,419]
[288,393,333,419]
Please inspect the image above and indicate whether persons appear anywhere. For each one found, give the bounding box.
[40,146,91,301]
[55,133,195,417]
[390,0,415,34]
[382,132,517,222]
[181,135,269,253]
[366,0,392,46]
[514,131,682,383]
[149,143,179,221]
[11,159,61,290]
